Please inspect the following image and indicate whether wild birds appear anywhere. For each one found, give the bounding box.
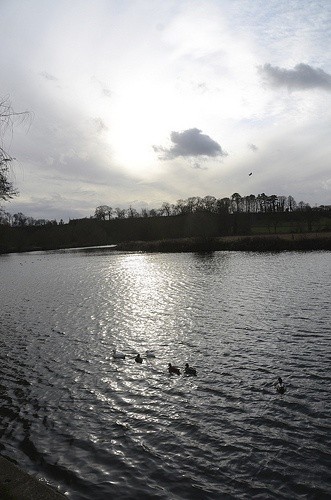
[135,354,143,363]
[248,172,252,176]
[145,350,155,357]
[185,363,196,373]
[167,363,180,374]
[275,377,287,393]
[111,349,125,359]
